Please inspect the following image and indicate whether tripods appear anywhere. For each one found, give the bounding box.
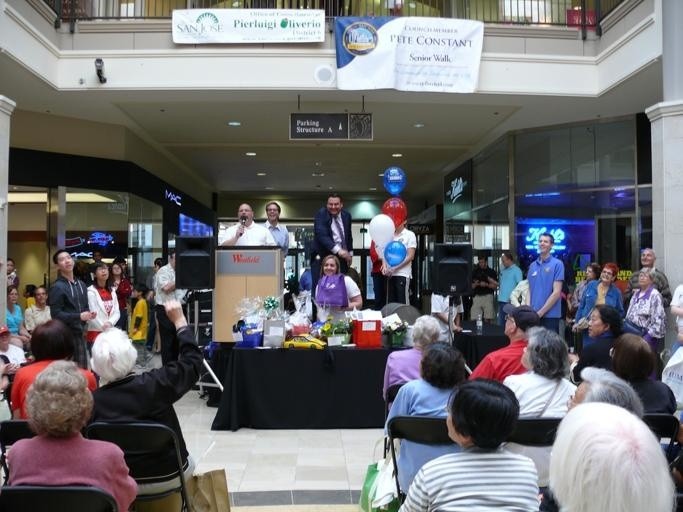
[177,292,224,398]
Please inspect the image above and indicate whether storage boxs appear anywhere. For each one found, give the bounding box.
[352,320,382,346]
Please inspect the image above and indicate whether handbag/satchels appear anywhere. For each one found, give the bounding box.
[572,317,588,333]
[186,469,231,512]
[371,458,401,508]
[358,464,401,512]
[316,274,347,308]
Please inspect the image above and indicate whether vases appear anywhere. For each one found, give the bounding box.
[325,334,351,344]
[387,332,402,345]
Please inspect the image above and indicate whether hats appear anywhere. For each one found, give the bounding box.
[0,324,9,334]
[502,304,540,330]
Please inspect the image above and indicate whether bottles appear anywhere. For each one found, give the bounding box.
[475,314,482,335]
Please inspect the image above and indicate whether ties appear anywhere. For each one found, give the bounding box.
[333,216,347,250]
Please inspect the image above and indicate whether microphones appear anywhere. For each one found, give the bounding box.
[239,216,246,238]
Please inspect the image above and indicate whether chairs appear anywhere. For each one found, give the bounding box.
[1,420,38,487]
[1,486,117,512]
[84,422,189,512]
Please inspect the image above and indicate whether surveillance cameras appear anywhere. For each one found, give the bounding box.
[94,58,104,71]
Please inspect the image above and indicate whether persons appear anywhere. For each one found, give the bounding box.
[537,366,646,512]
[369,239,386,310]
[6,359,138,512]
[263,201,290,258]
[313,254,363,322]
[388,218,418,307]
[82,299,203,494]
[430,291,464,347]
[0,249,191,419]
[381,340,467,499]
[379,314,443,414]
[546,402,677,512]
[468,230,682,423]
[297,269,312,303]
[222,202,276,248]
[312,191,355,304]
[396,376,540,512]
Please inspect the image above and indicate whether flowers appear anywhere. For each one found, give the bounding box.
[317,315,351,336]
[383,318,409,335]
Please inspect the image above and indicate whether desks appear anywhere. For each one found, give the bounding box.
[210,338,410,432]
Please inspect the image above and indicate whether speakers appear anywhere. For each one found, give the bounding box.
[434,244,473,298]
[175,237,215,290]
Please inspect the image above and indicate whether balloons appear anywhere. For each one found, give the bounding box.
[368,214,396,250]
[381,166,406,196]
[383,241,407,267]
[382,198,407,230]
